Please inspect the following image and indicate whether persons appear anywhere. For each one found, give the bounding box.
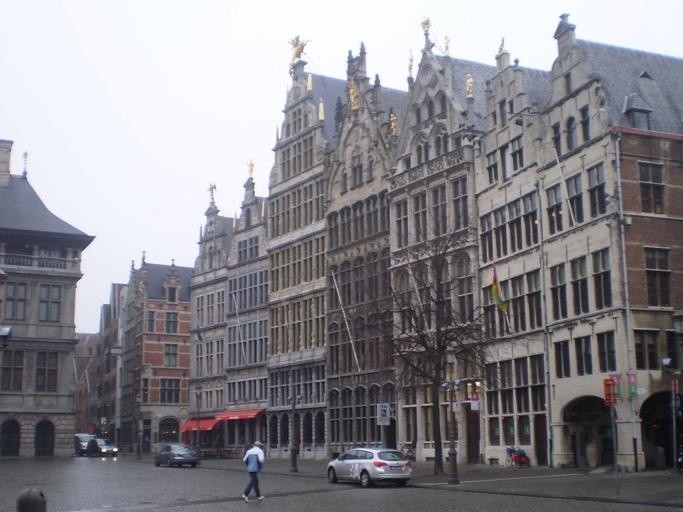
[206,183,218,203]
[246,158,254,179]
[240,441,266,504]
[15,486,47,512]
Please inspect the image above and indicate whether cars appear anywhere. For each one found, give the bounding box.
[89,434,98,439]
[326,447,415,488]
[154,444,199,467]
[87,437,118,457]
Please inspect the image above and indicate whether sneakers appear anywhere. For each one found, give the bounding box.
[257,495,265,501]
[241,493,251,503]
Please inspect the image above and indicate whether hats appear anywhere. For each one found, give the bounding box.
[254,440,265,447]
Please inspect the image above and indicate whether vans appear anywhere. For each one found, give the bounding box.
[73,433,92,456]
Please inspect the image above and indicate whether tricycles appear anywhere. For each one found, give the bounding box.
[505,448,529,467]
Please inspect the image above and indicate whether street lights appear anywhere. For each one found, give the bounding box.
[194,386,203,452]
[132,389,143,457]
[442,379,460,487]
[661,354,683,476]
[287,395,304,474]
